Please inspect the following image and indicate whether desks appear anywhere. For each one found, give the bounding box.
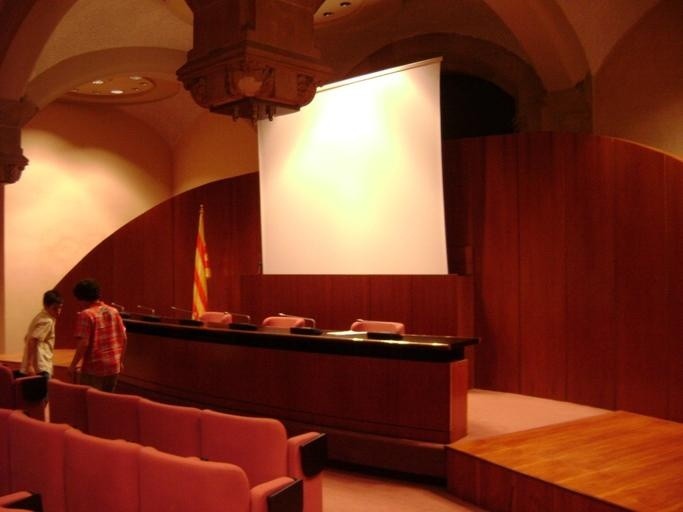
[119,316,478,445]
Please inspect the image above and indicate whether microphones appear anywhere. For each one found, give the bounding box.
[224,311,250,323]
[171,306,199,320]
[356,318,369,336]
[136,305,155,315]
[278,312,315,328]
[112,303,124,311]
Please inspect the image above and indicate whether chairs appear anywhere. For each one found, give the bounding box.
[351,321,405,334]
[201,312,232,325]
[137,445,304,511]
[202,410,327,511]
[9,410,68,511]
[86,389,135,445]
[1,364,49,422]
[64,426,140,510]
[136,396,201,460]
[262,317,305,329]
[48,378,90,435]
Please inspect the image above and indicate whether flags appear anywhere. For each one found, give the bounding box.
[191,214,212,320]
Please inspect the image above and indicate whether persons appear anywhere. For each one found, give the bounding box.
[20,291,62,376]
[66,279,128,391]
